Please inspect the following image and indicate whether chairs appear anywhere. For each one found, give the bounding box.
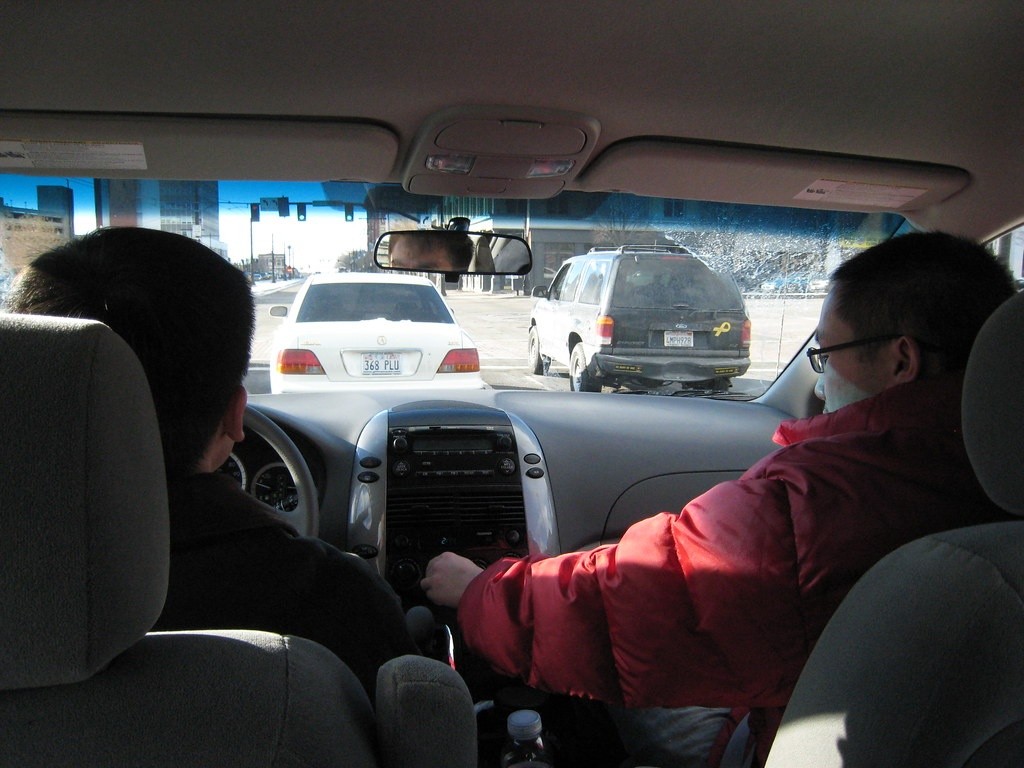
[762,289,1024,768]
[0,314,478,768]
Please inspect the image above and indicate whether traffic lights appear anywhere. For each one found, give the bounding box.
[345,203,355,223]
[278,197,290,217]
[251,203,261,223]
[298,203,307,222]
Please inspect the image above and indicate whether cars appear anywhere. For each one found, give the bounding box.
[267,272,483,393]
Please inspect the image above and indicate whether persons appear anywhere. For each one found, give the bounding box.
[388,232,475,271]
[420,228,1018,768]
[4,225,407,711]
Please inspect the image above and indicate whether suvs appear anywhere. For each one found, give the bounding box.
[530,245,748,398]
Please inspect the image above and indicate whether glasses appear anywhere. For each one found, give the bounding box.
[807,333,941,374]
